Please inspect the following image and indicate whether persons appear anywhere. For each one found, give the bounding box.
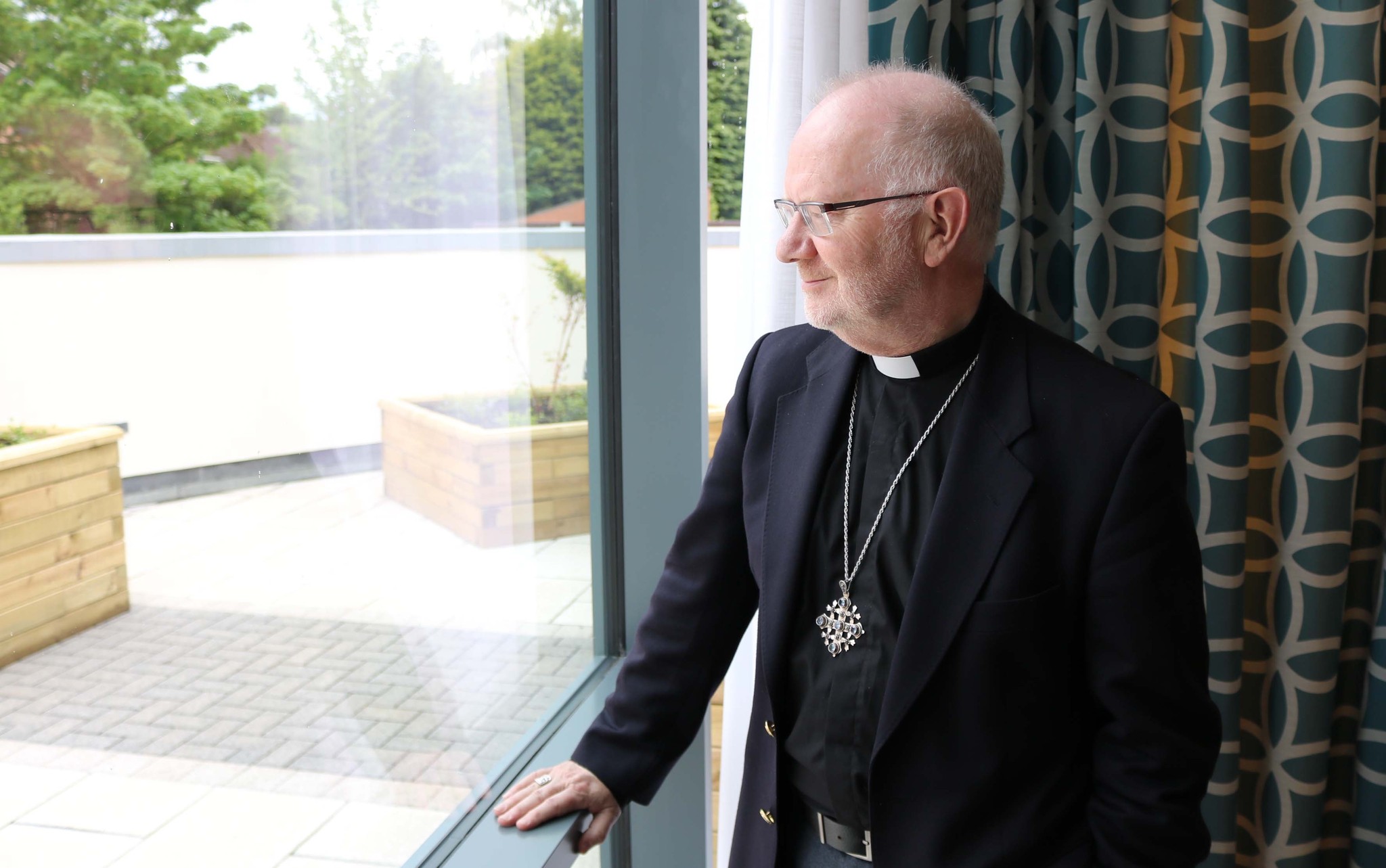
[493,70,1223,868]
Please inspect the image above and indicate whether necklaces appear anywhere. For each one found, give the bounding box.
[816,353,979,658]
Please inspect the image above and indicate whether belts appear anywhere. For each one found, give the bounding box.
[787,782,872,862]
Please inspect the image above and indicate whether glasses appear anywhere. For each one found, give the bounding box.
[773,189,938,237]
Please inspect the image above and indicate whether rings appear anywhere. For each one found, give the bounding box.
[535,775,552,786]
